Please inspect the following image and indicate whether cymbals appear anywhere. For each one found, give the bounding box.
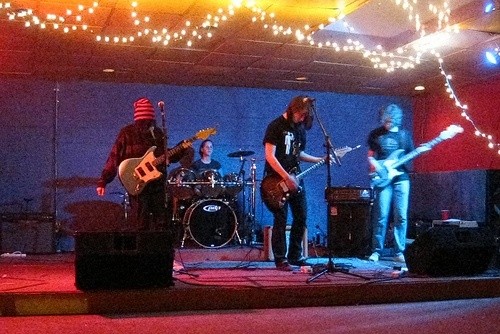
[227,150,255,158]
[245,177,263,181]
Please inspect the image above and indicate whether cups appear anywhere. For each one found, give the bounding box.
[441,210,450,220]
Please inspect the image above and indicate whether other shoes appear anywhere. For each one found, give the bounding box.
[369,252,381,262]
[394,252,406,263]
[277,260,296,270]
[288,260,313,266]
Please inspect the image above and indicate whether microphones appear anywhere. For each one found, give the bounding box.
[158,101,165,107]
[303,98,315,103]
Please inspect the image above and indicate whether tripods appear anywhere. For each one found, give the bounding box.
[306,104,371,283]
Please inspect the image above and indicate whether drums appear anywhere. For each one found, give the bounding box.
[223,172,243,194]
[183,198,239,249]
[167,168,196,200]
[197,169,223,198]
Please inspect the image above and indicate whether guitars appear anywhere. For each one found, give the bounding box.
[259,145,353,213]
[368,124,464,190]
[117,127,217,197]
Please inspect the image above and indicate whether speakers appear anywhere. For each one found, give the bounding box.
[326,202,375,259]
[402,169,500,278]
[73,231,173,289]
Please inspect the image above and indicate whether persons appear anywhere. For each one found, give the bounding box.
[96,98,193,231]
[367,104,416,263]
[263,94,335,271]
[188,139,223,178]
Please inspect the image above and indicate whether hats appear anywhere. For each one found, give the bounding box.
[133,98,158,121]
